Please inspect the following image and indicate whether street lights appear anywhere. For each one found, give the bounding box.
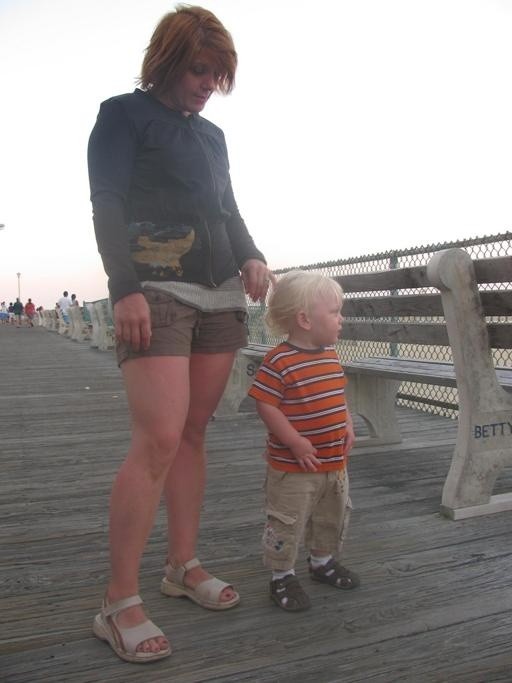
[0,223,4,230]
[17,270,21,302]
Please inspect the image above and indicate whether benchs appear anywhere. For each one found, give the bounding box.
[32,293,276,422]
[311,253,511,521]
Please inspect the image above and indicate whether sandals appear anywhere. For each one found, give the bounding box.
[92,592,172,663]
[307,556,361,590]
[159,559,240,610]
[269,574,312,611]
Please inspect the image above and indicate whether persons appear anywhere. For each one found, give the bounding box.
[248,270,362,614]
[86,4,278,665]
[54,290,78,324]
[1,298,47,329]
